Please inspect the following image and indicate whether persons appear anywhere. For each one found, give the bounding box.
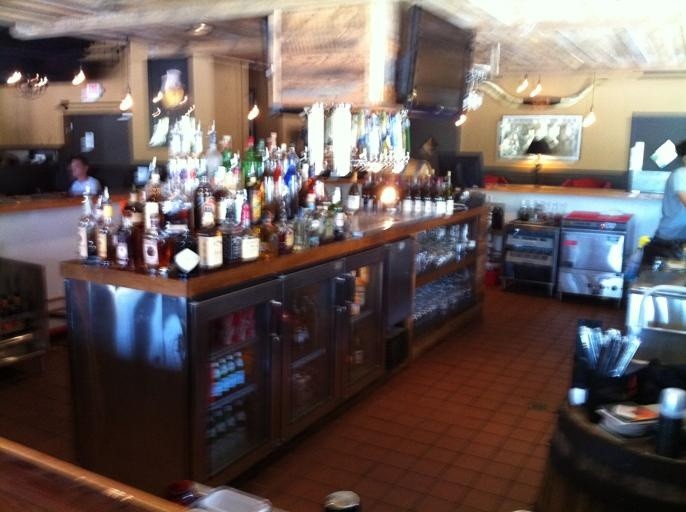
[639,140,686,271]
[66,155,102,193]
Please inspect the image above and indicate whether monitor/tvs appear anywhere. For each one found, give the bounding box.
[396,4,473,114]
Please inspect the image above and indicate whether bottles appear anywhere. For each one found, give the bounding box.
[516,198,562,228]
[349,170,458,216]
[209,352,248,400]
[413,268,477,334]
[164,68,184,106]
[206,398,256,461]
[78,112,345,279]
[412,224,475,272]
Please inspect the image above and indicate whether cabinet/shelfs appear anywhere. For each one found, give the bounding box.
[415,254,477,337]
[505,224,557,292]
[67,246,386,499]
